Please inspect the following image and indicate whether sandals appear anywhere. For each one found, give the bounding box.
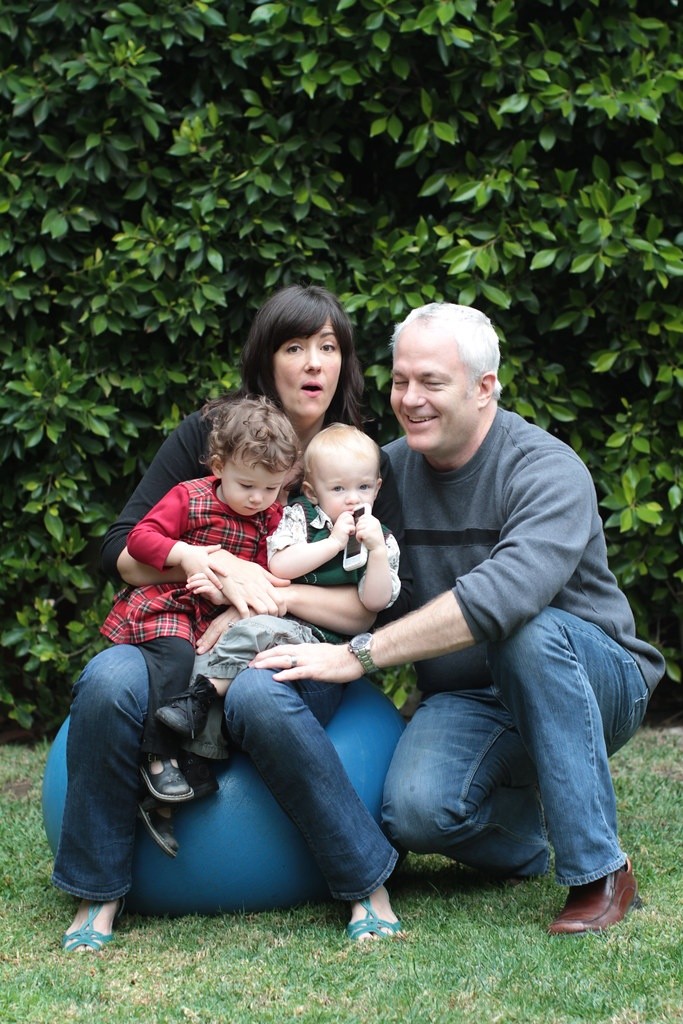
[60,895,127,959]
[347,884,402,947]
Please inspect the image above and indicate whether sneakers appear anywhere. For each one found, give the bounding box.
[177,751,219,800]
[154,674,219,740]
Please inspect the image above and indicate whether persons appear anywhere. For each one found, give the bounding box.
[155,422,402,796]
[248,305,669,932]
[61,285,417,951]
[100,400,299,859]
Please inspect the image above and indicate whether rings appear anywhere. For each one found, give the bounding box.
[228,622,235,627]
[290,655,296,667]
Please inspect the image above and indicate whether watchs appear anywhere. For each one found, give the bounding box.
[348,633,378,674]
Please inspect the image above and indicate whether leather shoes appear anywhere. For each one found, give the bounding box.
[548,857,640,937]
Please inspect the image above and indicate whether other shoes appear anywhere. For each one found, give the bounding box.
[137,795,180,858]
[139,751,194,803]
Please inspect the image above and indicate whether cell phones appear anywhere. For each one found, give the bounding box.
[343,503,372,571]
